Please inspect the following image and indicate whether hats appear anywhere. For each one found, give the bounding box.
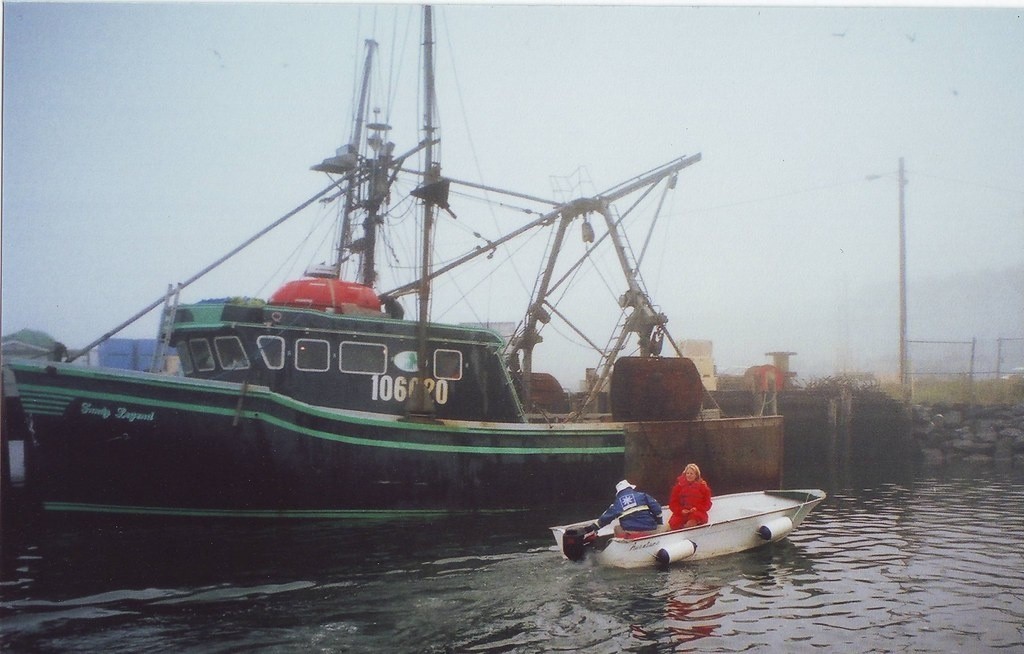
[615,479,636,497]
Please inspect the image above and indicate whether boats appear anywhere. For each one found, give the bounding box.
[1,3,722,558]
[550,488,826,568]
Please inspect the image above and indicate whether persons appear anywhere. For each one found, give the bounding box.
[591,479,663,539]
[669,464,713,529]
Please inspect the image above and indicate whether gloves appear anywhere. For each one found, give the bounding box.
[584,524,600,533]
[656,517,663,524]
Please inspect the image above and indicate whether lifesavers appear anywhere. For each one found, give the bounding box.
[756,364,784,392]
